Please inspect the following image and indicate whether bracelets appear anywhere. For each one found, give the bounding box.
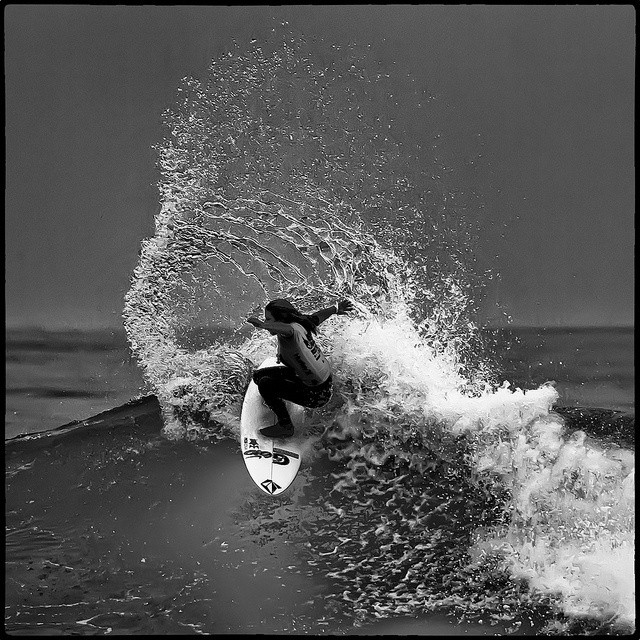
[333,304,339,314]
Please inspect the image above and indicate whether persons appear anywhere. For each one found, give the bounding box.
[245,298,354,439]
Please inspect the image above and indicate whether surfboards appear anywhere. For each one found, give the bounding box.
[240,356,305,496]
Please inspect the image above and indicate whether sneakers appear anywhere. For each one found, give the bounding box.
[260,423,295,438]
